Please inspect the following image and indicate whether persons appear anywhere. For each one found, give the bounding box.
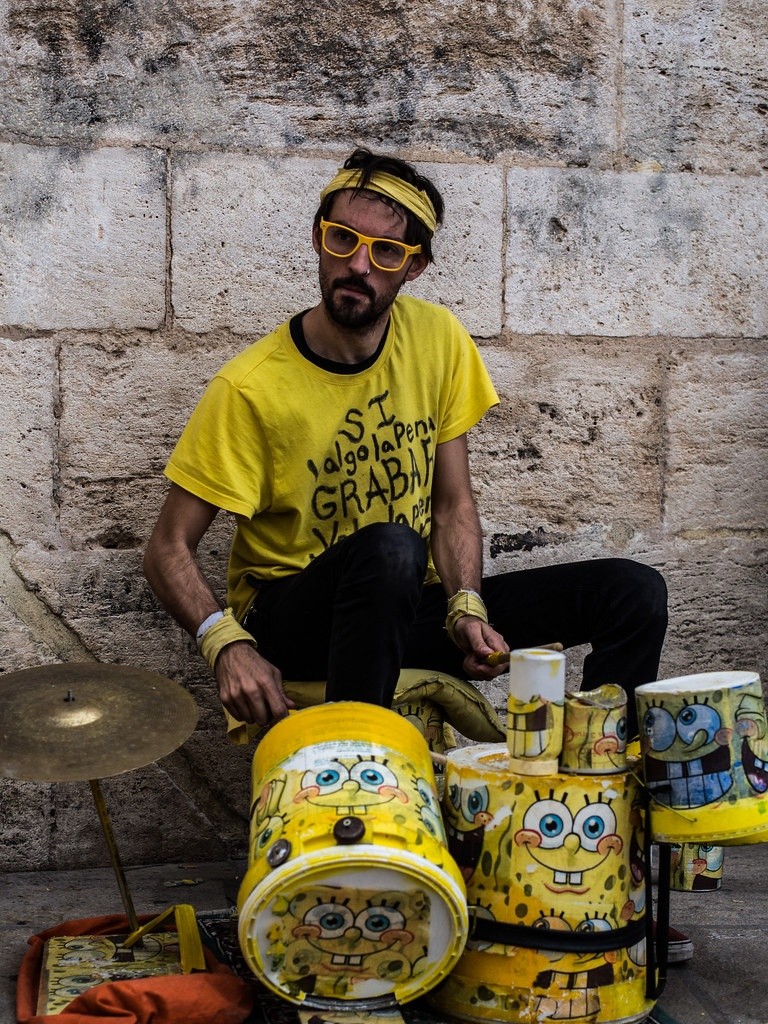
[144,145,699,967]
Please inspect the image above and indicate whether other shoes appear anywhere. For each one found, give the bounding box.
[654,921,694,962]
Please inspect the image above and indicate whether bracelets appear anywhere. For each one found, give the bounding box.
[445,588,490,649]
[193,607,257,670]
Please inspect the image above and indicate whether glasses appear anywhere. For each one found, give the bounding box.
[320,216,421,271]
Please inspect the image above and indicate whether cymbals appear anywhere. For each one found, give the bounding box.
[0,660,202,785]
